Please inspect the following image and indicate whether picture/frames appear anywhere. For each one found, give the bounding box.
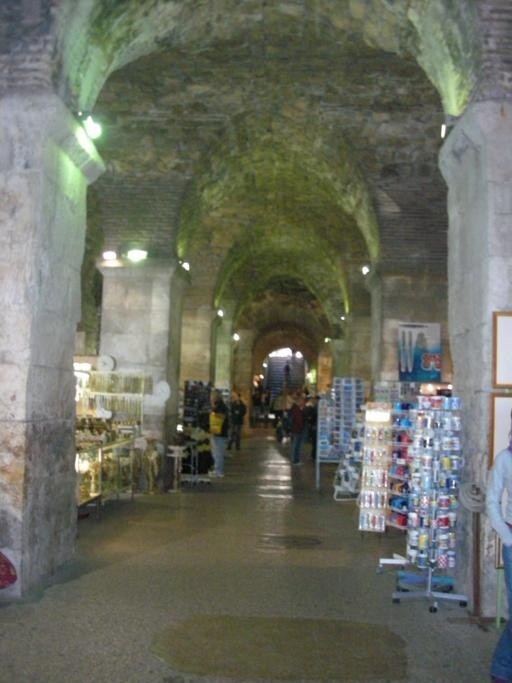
[488,393,512,472]
[493,312,512,389]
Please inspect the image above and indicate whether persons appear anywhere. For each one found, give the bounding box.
[484,407,512,683]
[207,388,247,479]
[252,383,320,466]
